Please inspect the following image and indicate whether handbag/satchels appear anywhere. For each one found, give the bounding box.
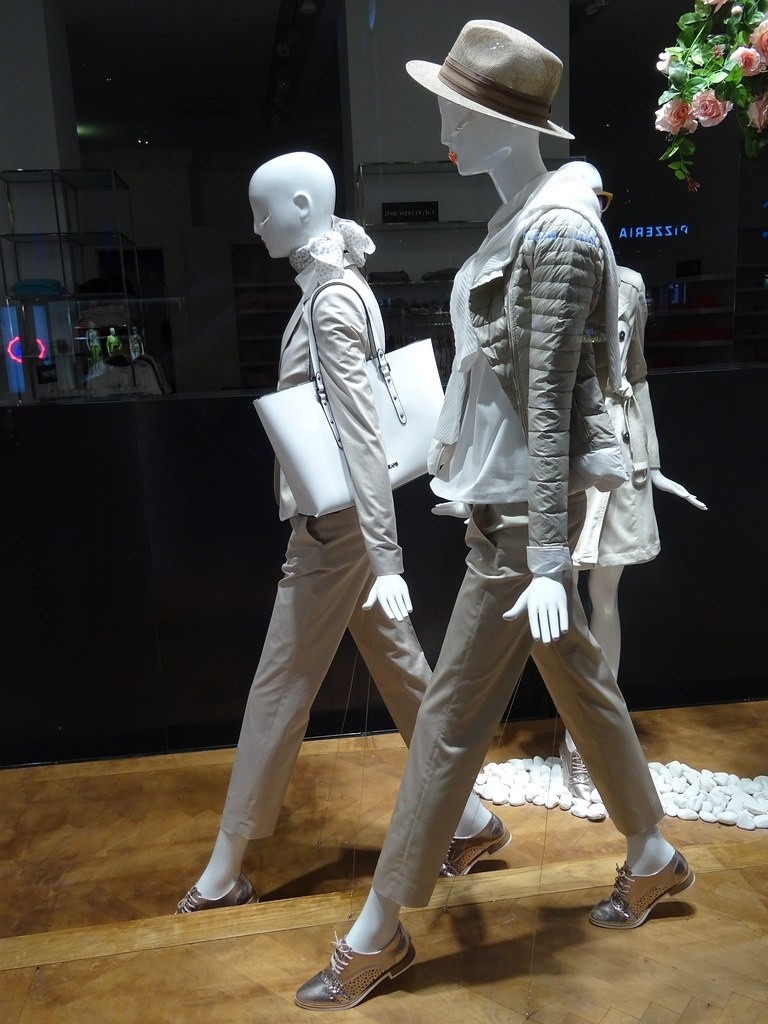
[251,280,446,517]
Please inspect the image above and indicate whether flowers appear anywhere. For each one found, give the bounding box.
[653,0,768,193]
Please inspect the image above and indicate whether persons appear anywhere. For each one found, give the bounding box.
[172,149,513,918]
[128,326,145,361]
[292,16,710,1016]
[86,321,102,373]
[106,327,123,358]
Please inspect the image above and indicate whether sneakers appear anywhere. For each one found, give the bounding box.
[589,850,696,930]
[171,872,259,915]
[558,738,596,804]
[296,919,416,1010]
[438,811,511,878]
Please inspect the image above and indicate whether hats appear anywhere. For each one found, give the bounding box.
[405,20,575,141]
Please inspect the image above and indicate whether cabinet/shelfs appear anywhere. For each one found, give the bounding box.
[228,239,300,388]
[645,272,734,349]
[0,169,148,394]
[735,220,768,341]
[357,157,582,392]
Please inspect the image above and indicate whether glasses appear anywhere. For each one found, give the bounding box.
[594,192,613,212]
